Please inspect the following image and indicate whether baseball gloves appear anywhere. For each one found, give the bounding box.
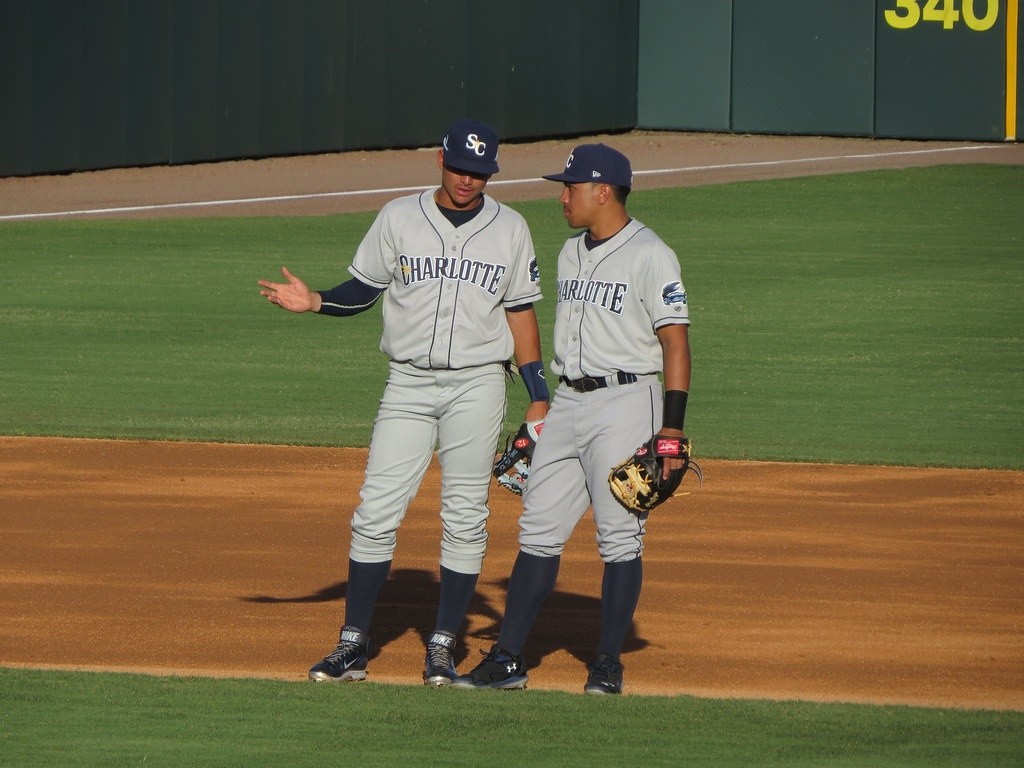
[493,419,544,496]
[607,432,689,513]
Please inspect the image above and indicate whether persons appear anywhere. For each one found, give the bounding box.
[253,118,551,684]
[449,142,696,693]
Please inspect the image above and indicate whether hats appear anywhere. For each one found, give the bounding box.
[442,124,500,179]
[544,143,632,188]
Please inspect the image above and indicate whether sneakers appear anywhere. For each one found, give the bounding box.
[423,628,459,688]
[308,624,368,683]
[584,650,624,697]
[450,644,529,692]
